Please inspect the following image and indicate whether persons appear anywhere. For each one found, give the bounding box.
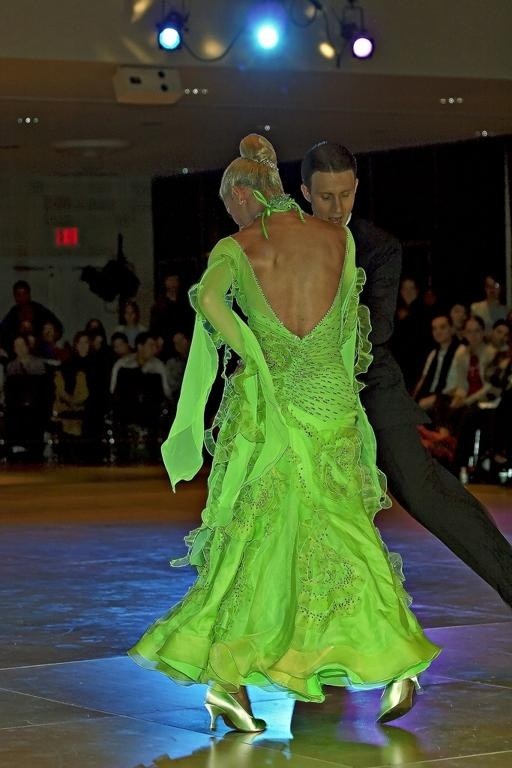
[125,129,444,734]
[394,271,511,487]
[290,123,510,621]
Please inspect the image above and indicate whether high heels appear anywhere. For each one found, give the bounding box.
[376,672,424,723]
[203,681,267,732]
